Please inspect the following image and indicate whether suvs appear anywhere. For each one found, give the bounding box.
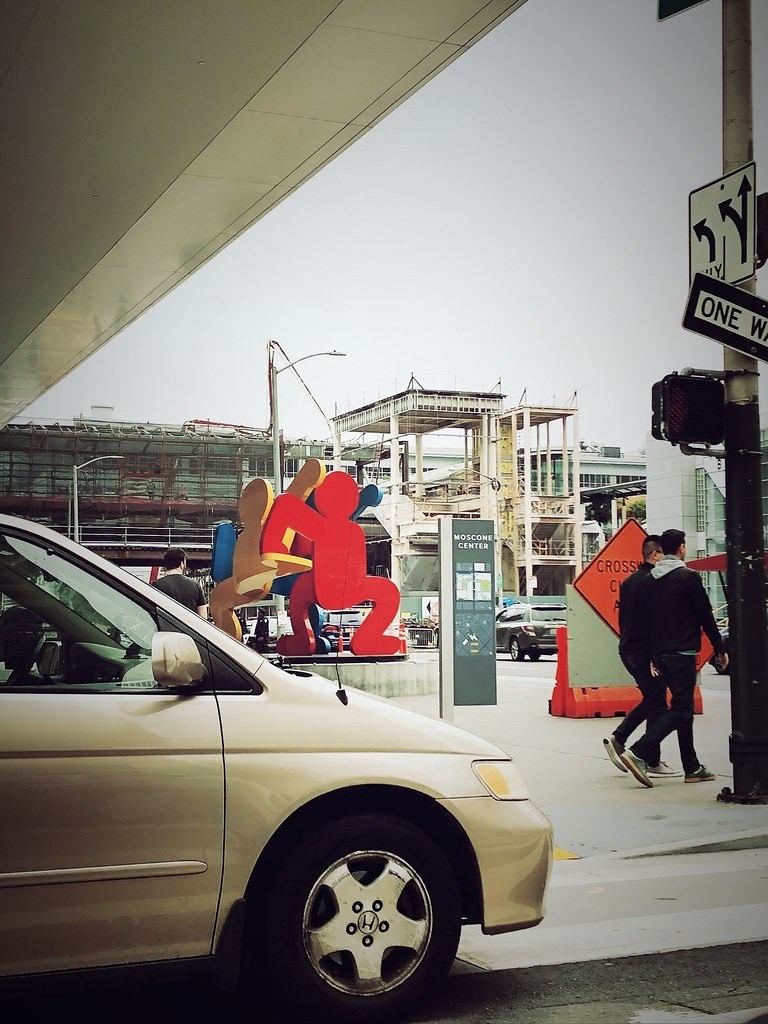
[322,610,365,652]
[496,602,566,662]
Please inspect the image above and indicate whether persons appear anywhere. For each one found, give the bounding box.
[603,529,729,787]
[151,548,207,621]
[254,608,270,653]
[233,609,247,643]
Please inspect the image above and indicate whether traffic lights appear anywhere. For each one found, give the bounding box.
[651,374,728,449]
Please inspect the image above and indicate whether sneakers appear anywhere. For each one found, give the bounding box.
[646,761,684,778]
[685,764,717,783]
[603,735,629,773]
[620,749,654,788]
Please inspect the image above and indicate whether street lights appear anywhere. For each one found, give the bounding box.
[72,454,125,547]
[270,350,346,500]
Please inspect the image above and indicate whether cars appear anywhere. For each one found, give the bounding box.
[708,626,732,676]
[0,509,553,1023]
